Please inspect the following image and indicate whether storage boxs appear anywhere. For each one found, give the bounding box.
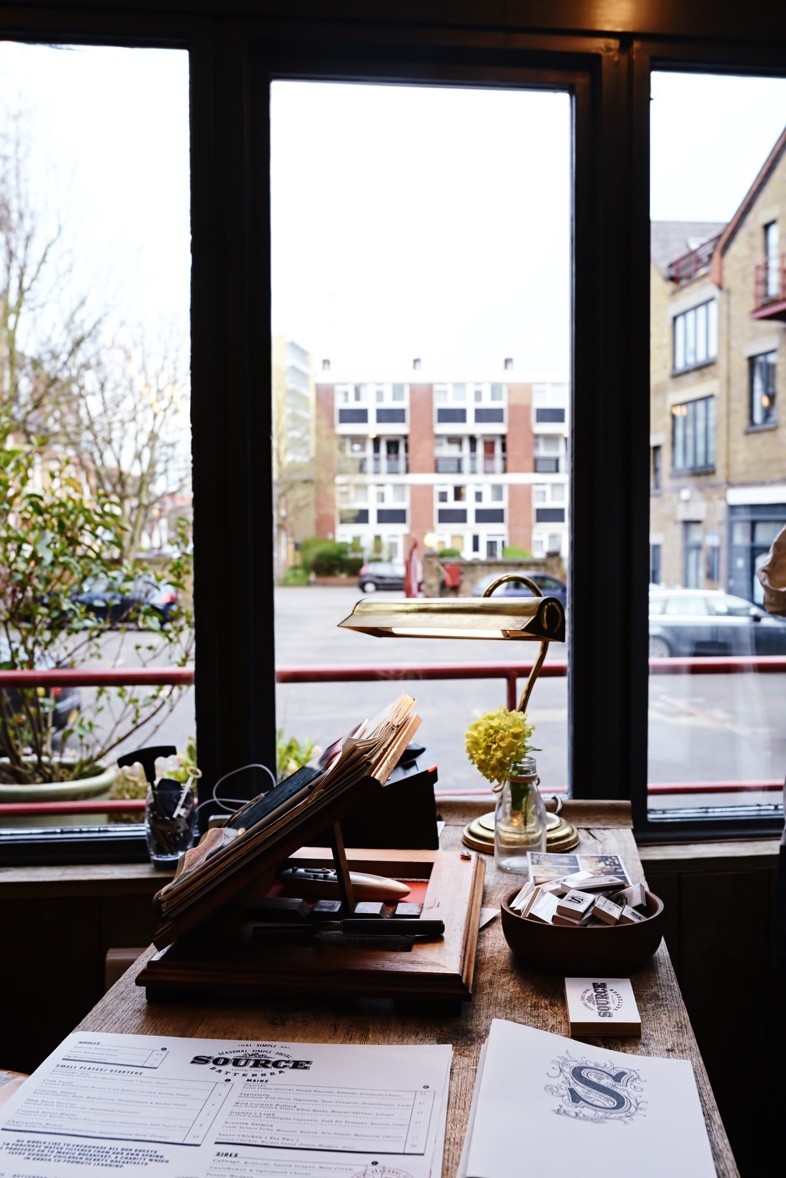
[509,870,647,1038]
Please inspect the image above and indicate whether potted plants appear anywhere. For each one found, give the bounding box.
[0,213,194,828]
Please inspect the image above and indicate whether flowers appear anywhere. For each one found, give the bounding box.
[465,709,542,833]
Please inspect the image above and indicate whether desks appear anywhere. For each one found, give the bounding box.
[0,795,744,1178]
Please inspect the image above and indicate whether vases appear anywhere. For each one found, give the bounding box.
[495,757,547,873]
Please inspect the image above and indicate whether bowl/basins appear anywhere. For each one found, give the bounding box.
[500,883,665,976]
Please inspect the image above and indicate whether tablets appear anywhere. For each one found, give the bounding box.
[158,716,371,902]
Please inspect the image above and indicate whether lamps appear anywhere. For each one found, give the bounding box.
[336,574,582,854]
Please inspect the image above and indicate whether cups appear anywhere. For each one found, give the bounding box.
[144,784,196,870]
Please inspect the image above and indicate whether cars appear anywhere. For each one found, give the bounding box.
[648,588,786,657]
[359,561,450,593]
[473,571,566,607]
[13,571,180,631]
